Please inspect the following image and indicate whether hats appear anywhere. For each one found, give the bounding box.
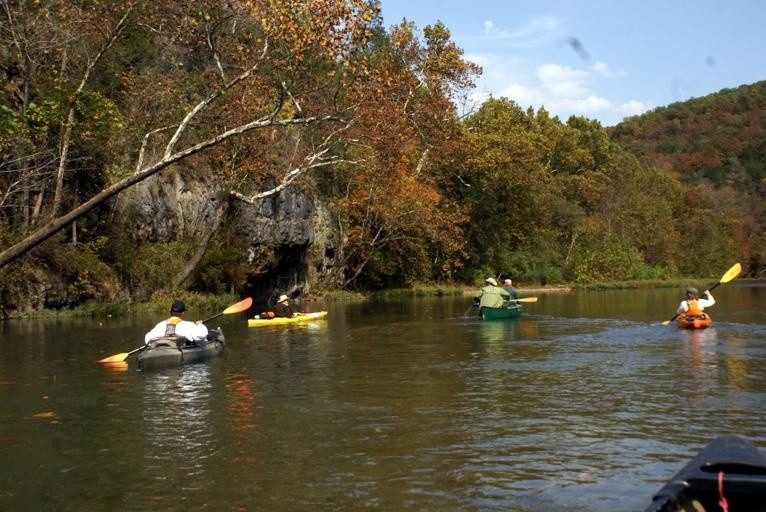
[277,295,290,303]
[171,301,188,313]
[486,277,497,286]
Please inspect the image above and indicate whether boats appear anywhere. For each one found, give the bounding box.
[677,309,712,329]
[247,311,327,328]
[480,306,520,321]
[137,328,224,369]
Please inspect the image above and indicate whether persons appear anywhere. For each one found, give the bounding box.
[677,287,716,317]
[273,294,296,318]
[144,301,209,346]
[471,278,520,311]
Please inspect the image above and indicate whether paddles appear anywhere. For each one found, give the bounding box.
[662,262,741,325]
[96,297,253,363]
[477,296,538,305]
